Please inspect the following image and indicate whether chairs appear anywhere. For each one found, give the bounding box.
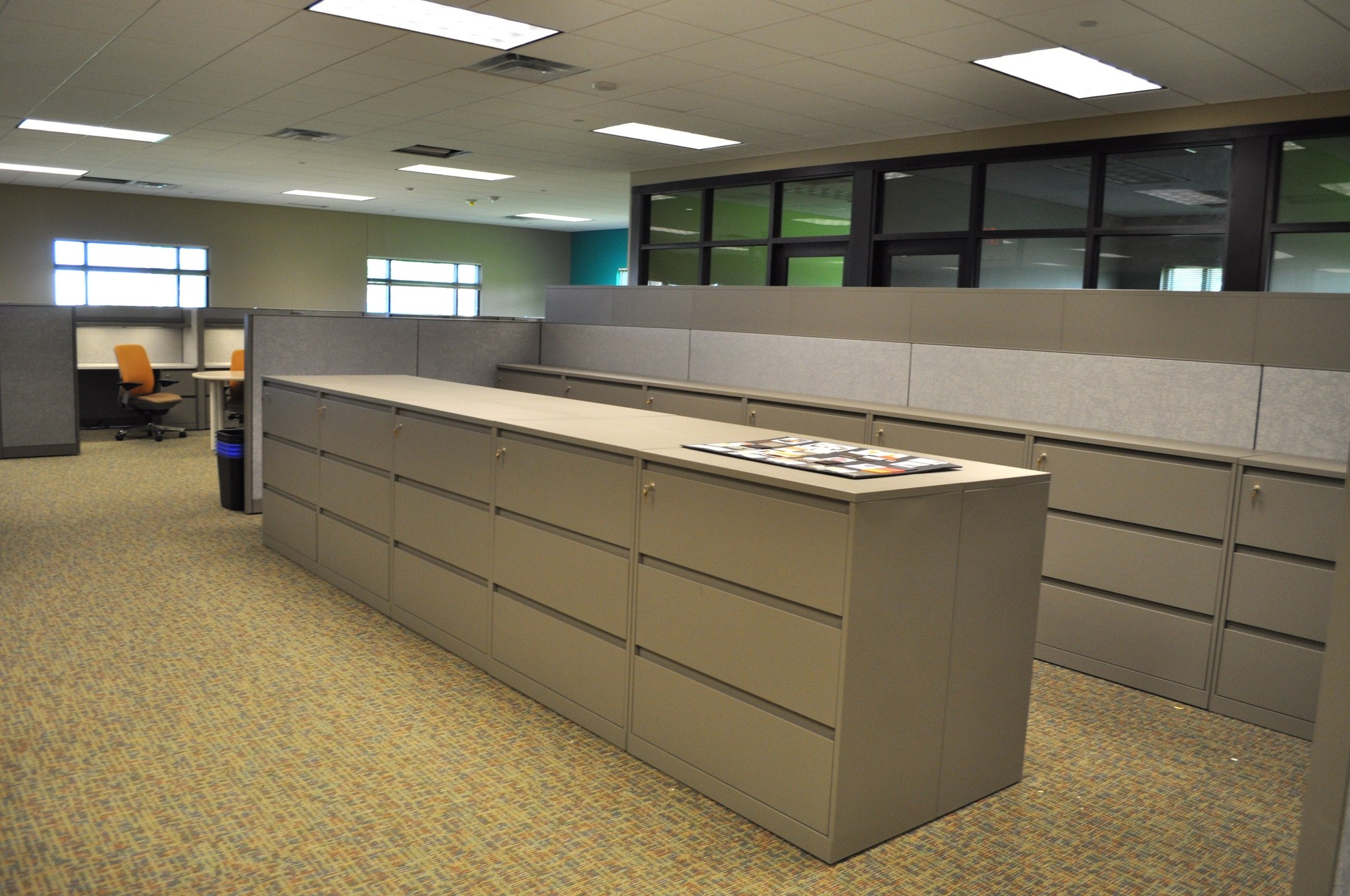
[223,349,245,429]
[114,344,187,442]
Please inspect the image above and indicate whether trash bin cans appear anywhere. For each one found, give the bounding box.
[214,428,245,511]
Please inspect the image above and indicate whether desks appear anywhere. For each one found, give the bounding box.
[205,362,231,368]
[191,371,245,450]
[76,363,196,369]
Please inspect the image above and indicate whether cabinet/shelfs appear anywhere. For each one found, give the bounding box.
[158,370,239,430]
[260,364,1347,866]
